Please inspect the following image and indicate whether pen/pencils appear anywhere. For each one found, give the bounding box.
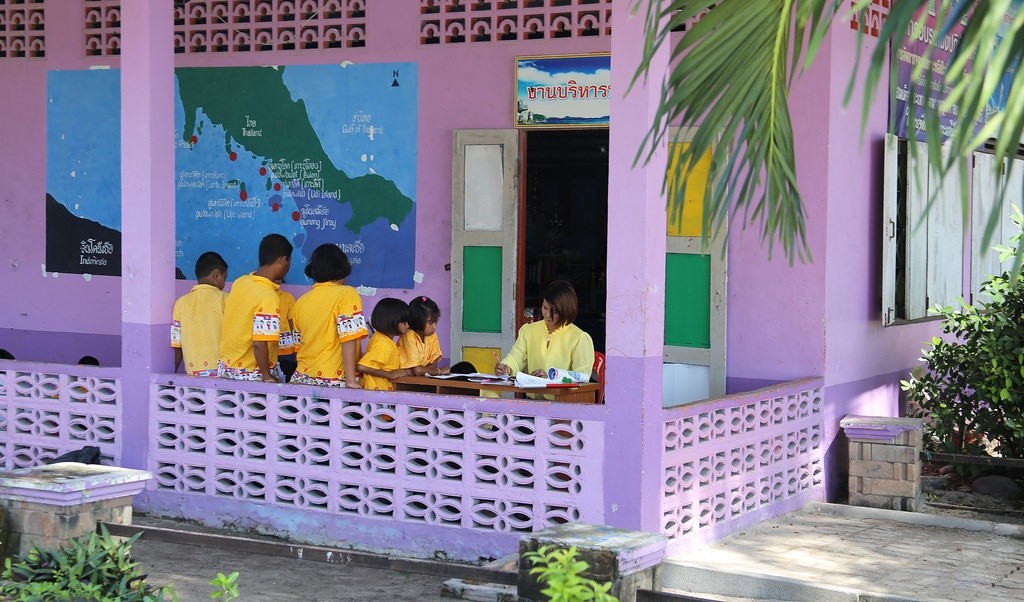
[493,351,510,378]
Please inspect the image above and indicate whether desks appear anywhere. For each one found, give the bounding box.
[388,374,601,523]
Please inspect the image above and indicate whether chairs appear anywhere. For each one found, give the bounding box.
[592,351,605,405]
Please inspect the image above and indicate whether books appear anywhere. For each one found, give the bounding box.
[426,367,591,388]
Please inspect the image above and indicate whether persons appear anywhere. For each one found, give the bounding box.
[495,279,596,400]
[52,355,100,402]
[396,296,451,377]
[170,233,368,388]
[356,298,412,420]
[0,349,15,374]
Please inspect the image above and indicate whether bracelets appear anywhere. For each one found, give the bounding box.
[436,368,441,375]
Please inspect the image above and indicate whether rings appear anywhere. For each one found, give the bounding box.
[539,370,542,373]
